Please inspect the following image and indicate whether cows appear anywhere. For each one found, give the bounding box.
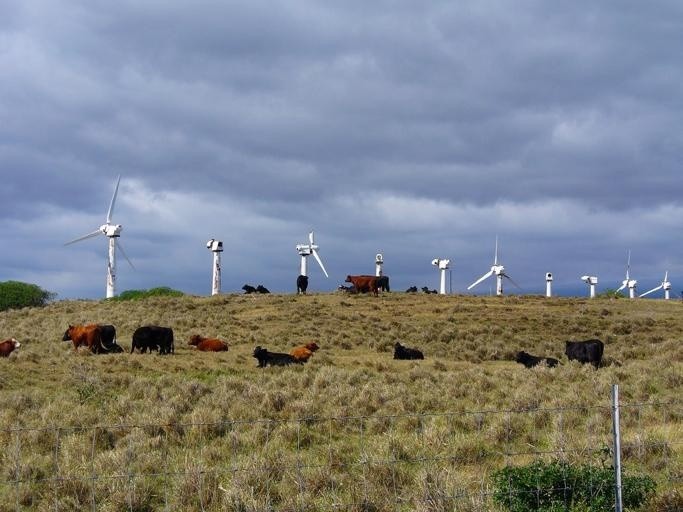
[296,274,308,295]
[393,341,424,360]
[241,284,271,294]
[0,336,21,357]
[565,339,604,371]
[515,351,564,369]
[251,342,320,367]
[405,285,437,294]
[61,323,227,355]
[336,273,390,297]
